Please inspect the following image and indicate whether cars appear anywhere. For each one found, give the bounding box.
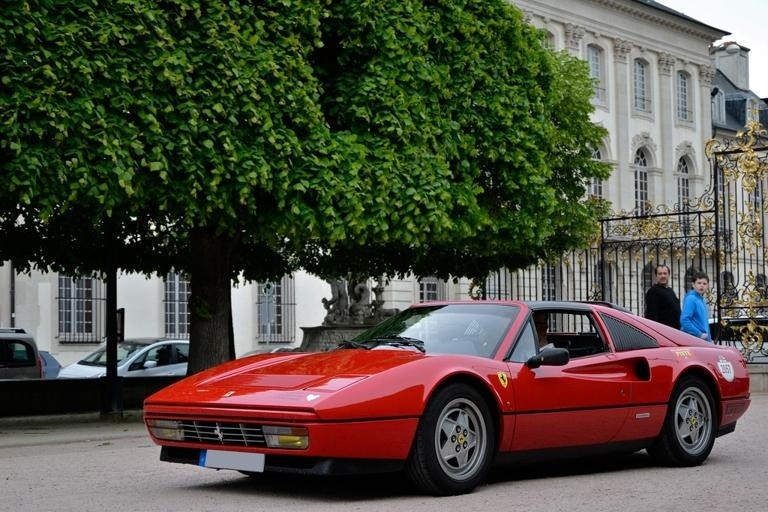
[54,336,190,378]
[14,348,62,380]
[238,345,301,358]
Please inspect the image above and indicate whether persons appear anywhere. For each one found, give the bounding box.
[529,314,560,352]
[644,265,681,330]
[679,272,712,344]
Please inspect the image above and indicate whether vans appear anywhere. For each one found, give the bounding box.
[0,327,47,380]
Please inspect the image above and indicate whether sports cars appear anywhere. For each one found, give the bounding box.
[138,297,757,501]
